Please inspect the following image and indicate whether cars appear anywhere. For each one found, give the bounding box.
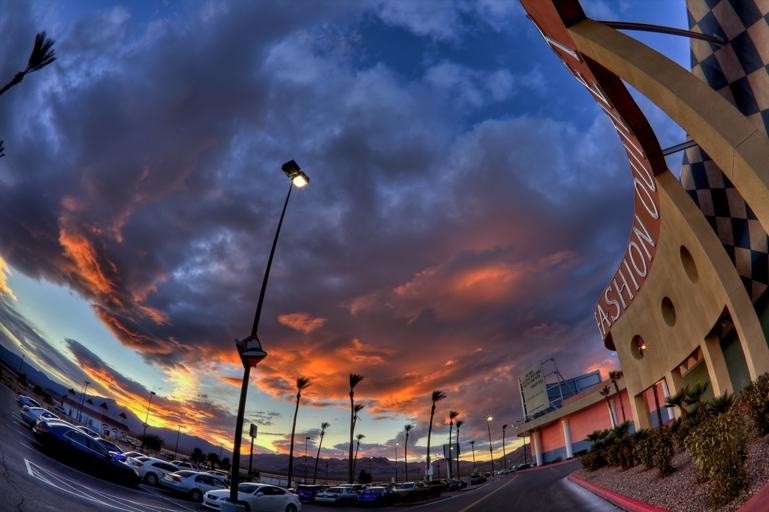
[470,463,531,484]
[15,394,466,512]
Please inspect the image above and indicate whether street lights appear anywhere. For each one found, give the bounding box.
[230,159,310,503]
[486,416,494,477]
